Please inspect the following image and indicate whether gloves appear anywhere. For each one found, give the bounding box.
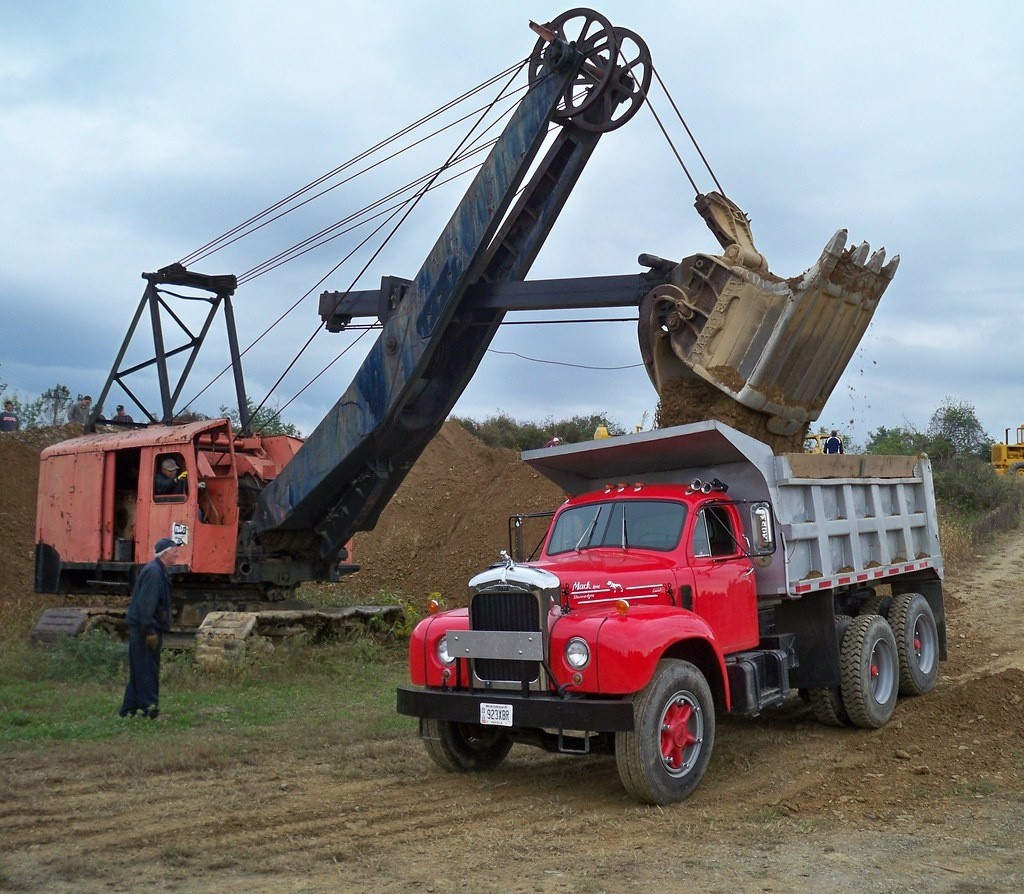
[147,634,159,651]
[178,471,188,480]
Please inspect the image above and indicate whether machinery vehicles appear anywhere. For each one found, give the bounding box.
[32,5,906,676]
[987,423,1024,482]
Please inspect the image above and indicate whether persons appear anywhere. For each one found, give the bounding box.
[119,540,182,718]
[0,400,19,432]
[544,437,570,448]
[823,429,844,453]
[110,405,134,422]
[154,459,204,522]
[89,405,107,425]
[67,396,92,424]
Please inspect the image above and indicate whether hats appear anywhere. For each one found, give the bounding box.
[162,459,180,471]
[117,405,124,411]
[154,538,183,553]
[84,396,92,401]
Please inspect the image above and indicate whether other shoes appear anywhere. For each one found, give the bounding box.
[155,714,171,722]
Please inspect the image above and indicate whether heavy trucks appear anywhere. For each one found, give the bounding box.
[396,421,950,807]
